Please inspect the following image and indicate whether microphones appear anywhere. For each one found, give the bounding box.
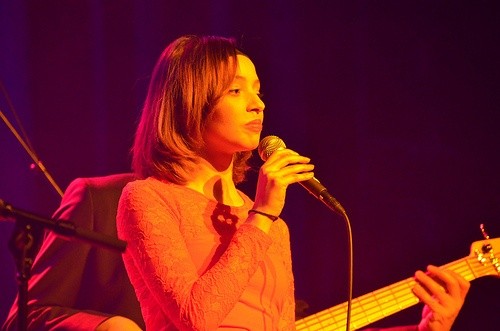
[258,136,345,215]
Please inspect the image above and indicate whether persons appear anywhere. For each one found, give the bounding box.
[0,168,148,331]
[115,34,472,331]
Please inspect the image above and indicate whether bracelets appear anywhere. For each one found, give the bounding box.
[247,210,278,223]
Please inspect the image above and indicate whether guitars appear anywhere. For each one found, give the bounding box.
[295,223,500,330]
